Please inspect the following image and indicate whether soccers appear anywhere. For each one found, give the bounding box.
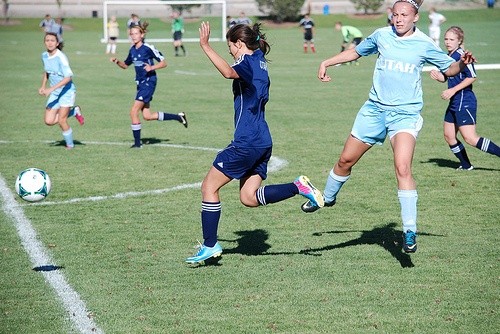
[15,168,51,202]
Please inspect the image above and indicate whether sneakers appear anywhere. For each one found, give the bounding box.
[293,174,325,208]
[74,106,84,125]
[458,164,474,170]
[131,144,143,149]
[186,238,224,265]
[301,194,337,213]
[402,228,418,253]
[177,112,187,128]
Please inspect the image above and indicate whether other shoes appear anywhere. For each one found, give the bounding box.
[344,62,350,65]
[356,60,359,65]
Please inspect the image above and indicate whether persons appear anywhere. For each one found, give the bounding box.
[38,32,84,149]
[226,12,252,31]
[39,14,64,52]
[426,8,447,49]
[297,0,478,254]
[427,26,499,170]
[110,22,189,149]
[334,22,364,66]
[298,13,316,53]
[170,13,186,57]
[182,19,325,267]
[386,7,394,26]
[105,13,141,55]
